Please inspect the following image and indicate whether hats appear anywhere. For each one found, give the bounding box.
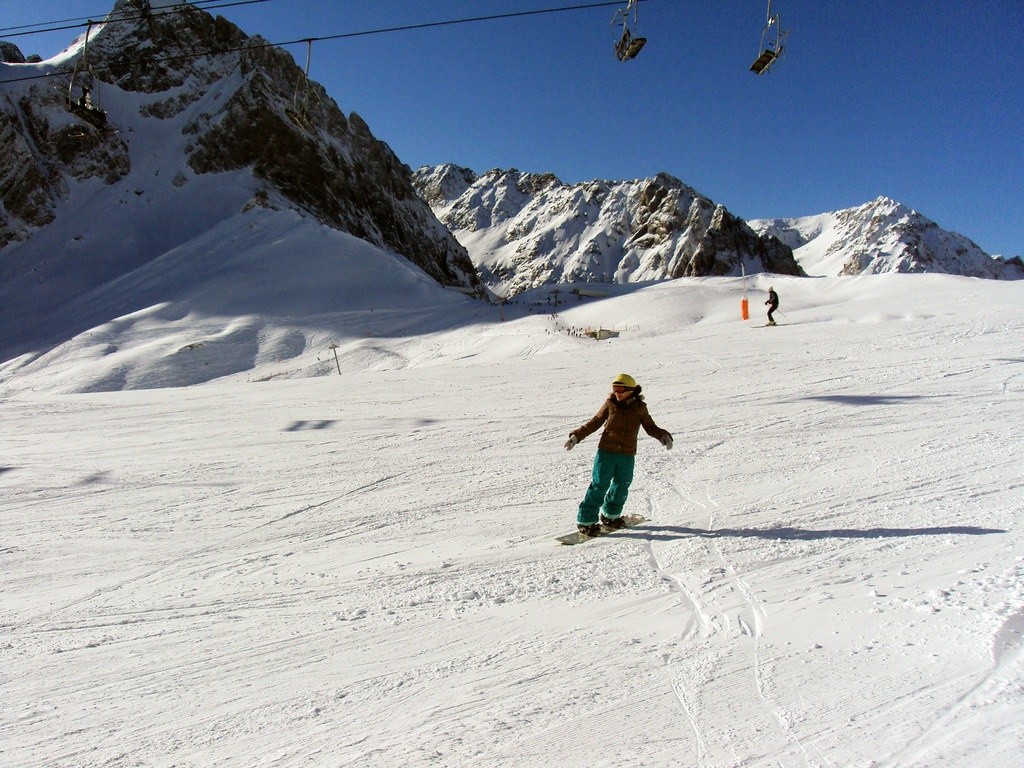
[611,373,637,387]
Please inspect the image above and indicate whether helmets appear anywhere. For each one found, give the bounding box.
[768,286,774,291]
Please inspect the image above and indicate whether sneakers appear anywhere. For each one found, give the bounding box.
[600,514,626,529]
[577,523,601,537]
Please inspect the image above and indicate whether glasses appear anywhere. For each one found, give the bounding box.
[612,387,634,394]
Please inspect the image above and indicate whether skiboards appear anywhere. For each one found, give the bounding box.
[751,323,787,328]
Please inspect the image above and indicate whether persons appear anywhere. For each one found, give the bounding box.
[563,373,673,536]
[765,286,778,325]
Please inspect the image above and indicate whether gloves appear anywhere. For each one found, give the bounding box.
[765,301,768,305]
[661,434,673,451]
[564,436,577,451]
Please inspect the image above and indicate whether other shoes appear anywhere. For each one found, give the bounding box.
[766,322,778,327]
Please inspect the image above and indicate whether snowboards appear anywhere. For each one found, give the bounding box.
[554,512,645,546]
